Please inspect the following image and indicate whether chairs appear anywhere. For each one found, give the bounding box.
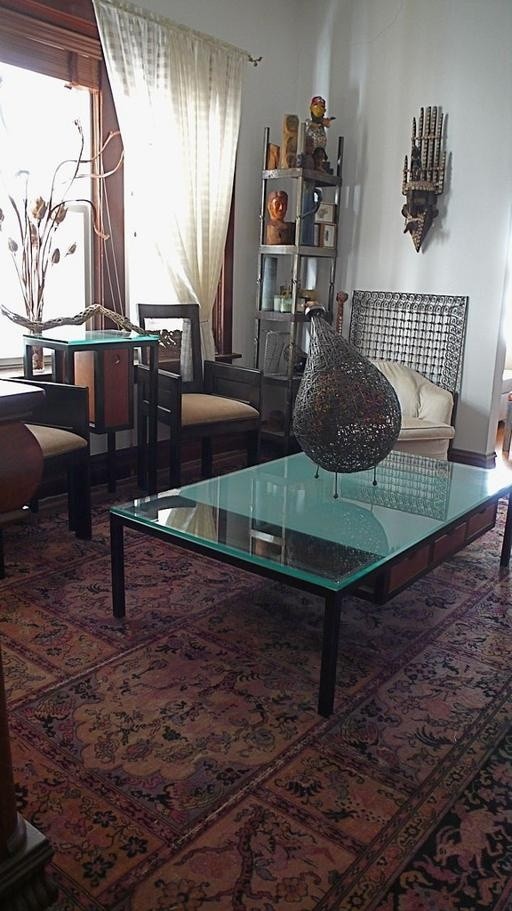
[0,376,90,542]
[366,356,453,462]
[131,302,266,491]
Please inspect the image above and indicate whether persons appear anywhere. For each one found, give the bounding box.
[264,191,296,245]
[305,95,333,171]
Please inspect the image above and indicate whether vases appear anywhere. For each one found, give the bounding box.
[27,330,45,372]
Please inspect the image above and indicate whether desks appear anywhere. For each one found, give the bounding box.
[23,329,162,497]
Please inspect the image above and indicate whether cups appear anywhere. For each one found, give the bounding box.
[273,294,305,313]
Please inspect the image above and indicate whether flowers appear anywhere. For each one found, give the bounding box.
[4,188,77,336]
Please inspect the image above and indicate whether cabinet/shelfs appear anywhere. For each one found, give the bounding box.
[251,127,344,465]
